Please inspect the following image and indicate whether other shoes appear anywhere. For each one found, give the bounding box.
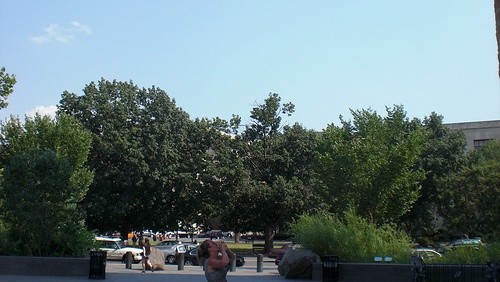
[142,270,145,273]
[151,266,154,272]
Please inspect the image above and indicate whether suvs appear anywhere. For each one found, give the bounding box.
[90,236,145,264]
[436,236,482,258]
[149,239,198,265]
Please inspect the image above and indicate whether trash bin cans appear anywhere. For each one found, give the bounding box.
[322,255,338,282]
[88,251,107,279]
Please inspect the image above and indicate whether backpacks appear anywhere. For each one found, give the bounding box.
[207,239,229,268]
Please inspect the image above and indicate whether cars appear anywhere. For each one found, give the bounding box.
[408,248,442,265]
[199,230,255,239]
[136,229,156,237]
[275,243,303,266]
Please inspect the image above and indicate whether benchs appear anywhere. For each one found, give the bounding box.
[245,239,254,243]
[411,254,500,282]
[253,244,266,252]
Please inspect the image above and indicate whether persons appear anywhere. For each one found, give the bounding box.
[138,233,143,247]
[197,239,235,282]
[142,238,154,273]
[132,235,136,247]
[168,233,172,238]
